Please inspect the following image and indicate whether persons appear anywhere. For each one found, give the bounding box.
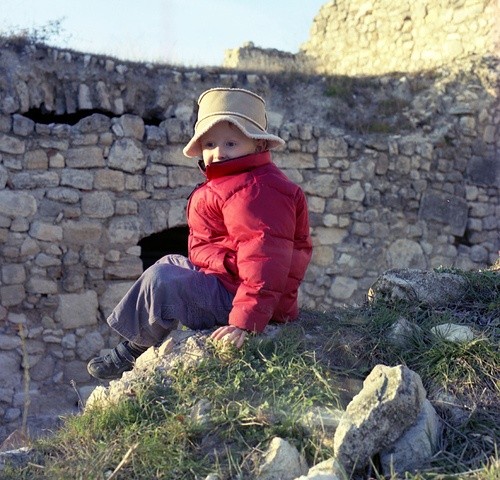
[87,87,313,381]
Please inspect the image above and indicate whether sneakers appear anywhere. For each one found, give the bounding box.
[87,340,147,381]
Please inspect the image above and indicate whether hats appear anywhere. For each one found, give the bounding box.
[182,87,286,159]
